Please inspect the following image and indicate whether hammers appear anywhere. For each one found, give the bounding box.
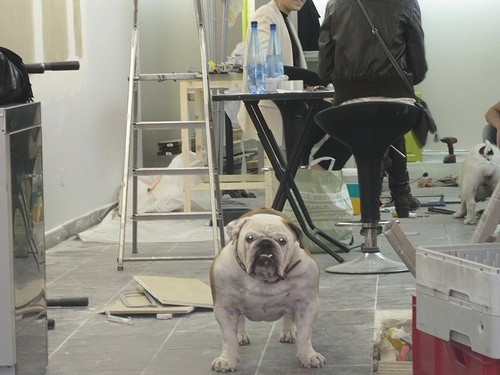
[440,137,458,154]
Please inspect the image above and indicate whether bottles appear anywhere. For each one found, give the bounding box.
[245,21,267,94]
[264,24,284,78]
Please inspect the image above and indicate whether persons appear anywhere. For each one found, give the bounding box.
[255,0,352,171]
[319,0,428,218]
[482,102,500,148]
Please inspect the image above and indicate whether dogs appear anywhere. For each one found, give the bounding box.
[209,207,327,372]
[452,139,500,224]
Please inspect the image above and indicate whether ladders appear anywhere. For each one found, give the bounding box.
[116,0,226,271]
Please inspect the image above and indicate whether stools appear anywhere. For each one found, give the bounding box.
[312,99,419,277]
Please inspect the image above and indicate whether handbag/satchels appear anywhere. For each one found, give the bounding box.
[412,99,438,149]
[0,47,34,107]
[265,157,357,253]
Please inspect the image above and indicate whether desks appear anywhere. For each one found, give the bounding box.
[212,88,353,264]
[178,80,304,217]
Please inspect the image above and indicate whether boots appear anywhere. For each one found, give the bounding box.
[388,136,421,218]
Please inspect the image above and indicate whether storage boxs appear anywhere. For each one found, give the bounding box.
[410,242,500,375]
